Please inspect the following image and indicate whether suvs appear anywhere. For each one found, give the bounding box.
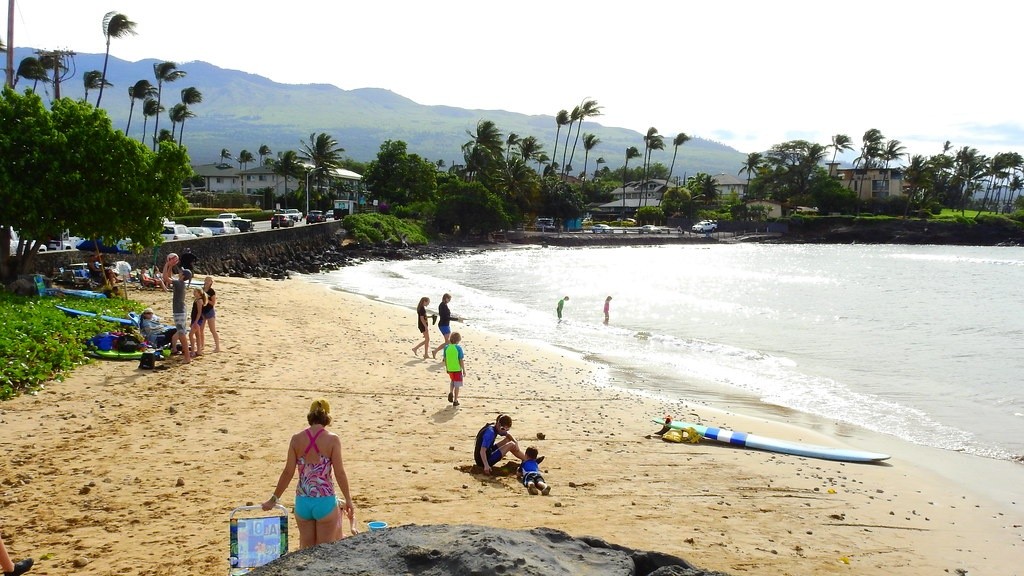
[117,212,255,254]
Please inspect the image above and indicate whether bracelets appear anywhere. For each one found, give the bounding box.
[271,495,278,502]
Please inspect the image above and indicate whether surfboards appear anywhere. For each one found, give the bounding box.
[651,416,893,462]
[53,303,178,330]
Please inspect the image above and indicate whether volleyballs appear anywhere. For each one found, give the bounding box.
[167,252,179,265]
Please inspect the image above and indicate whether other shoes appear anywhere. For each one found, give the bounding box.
[448,392,453,402]
[453,401,460,405]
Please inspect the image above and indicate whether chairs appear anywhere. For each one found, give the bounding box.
[149,267,172,289]
[228,503,289,576]
[136,268,162,291]
[62,270,76,282]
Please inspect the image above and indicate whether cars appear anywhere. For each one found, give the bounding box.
[325,209,335,219]
[640,224,663,234]
[306,210,326,225]
[591,223,614,234]
[22,235,86,253]
[286,209,303,222]
[625,217,637,227]
[270,208,294,229]
[692,221,718,234]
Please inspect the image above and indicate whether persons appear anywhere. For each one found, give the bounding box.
[141,267,169,292]
[412,297,432,358]
[181,247,197,277]
[104,287,120,298]
[87,254,124,286]
[163,257,192,365]
[140,309,183,355]
[516,445,551,495]
[38,264,54,288]
[189,286,208,356]
[62,267,76,289]
[443,331,466,406]
[473,413,545,473]
[153,266,169,288]
[261,398,355,549]
[556,295,569,319]
[432,293,463,359]
[603,296,612,325]
[200,276,220,353]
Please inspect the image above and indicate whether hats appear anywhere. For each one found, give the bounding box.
[145,308,152,314]
[182,267,191,280]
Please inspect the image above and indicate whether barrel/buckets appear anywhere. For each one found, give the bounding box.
[163,350,171,357]
[366,520,388,532]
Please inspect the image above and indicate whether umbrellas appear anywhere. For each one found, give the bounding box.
[77,238,133,253]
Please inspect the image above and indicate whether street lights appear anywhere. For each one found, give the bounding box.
[306,167,323,215]
